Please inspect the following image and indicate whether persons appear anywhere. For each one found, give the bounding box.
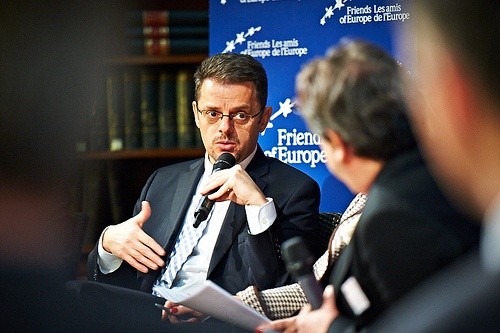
[37,53,320,333]
[256,39,482,333]
[360,0,500,333]
[162,37,368,328]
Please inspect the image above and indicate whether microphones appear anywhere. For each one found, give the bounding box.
[193,153,235,228]
[282,237,323,311]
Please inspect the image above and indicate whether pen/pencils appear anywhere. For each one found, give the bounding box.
[155,304,189,319]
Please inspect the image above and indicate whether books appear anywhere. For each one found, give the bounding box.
[69,163,127,257]
[66,70,204,148]
[125,5,209,54]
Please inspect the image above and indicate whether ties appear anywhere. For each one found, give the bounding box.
[152,168,215,298]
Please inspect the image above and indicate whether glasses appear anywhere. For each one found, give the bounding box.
[197,105,267,126]
